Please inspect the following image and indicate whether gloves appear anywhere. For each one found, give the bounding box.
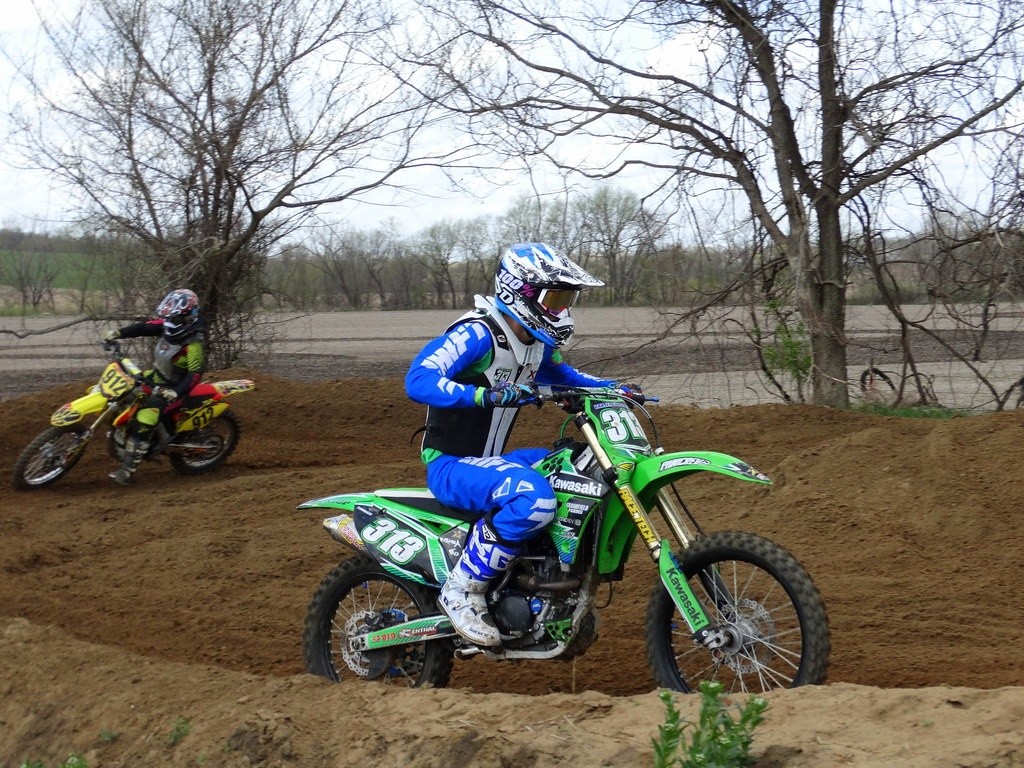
[162,389,178,403]
[102,329,121,341]
[481,380,536,409]
[616,382,644,409]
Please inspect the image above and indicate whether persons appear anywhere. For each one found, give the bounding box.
[405,243,645,647]
[105,289,205,486]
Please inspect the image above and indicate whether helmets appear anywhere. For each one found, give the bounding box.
[495,242,605,349]
[156,288,201,337]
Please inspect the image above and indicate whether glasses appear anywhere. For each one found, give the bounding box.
[498,264,580,319]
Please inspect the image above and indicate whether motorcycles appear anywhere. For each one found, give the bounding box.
[12,338,256,492]
[296,382,831,692]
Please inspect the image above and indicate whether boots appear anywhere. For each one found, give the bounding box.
[107,437,151,486]
[437,507,522,646]
